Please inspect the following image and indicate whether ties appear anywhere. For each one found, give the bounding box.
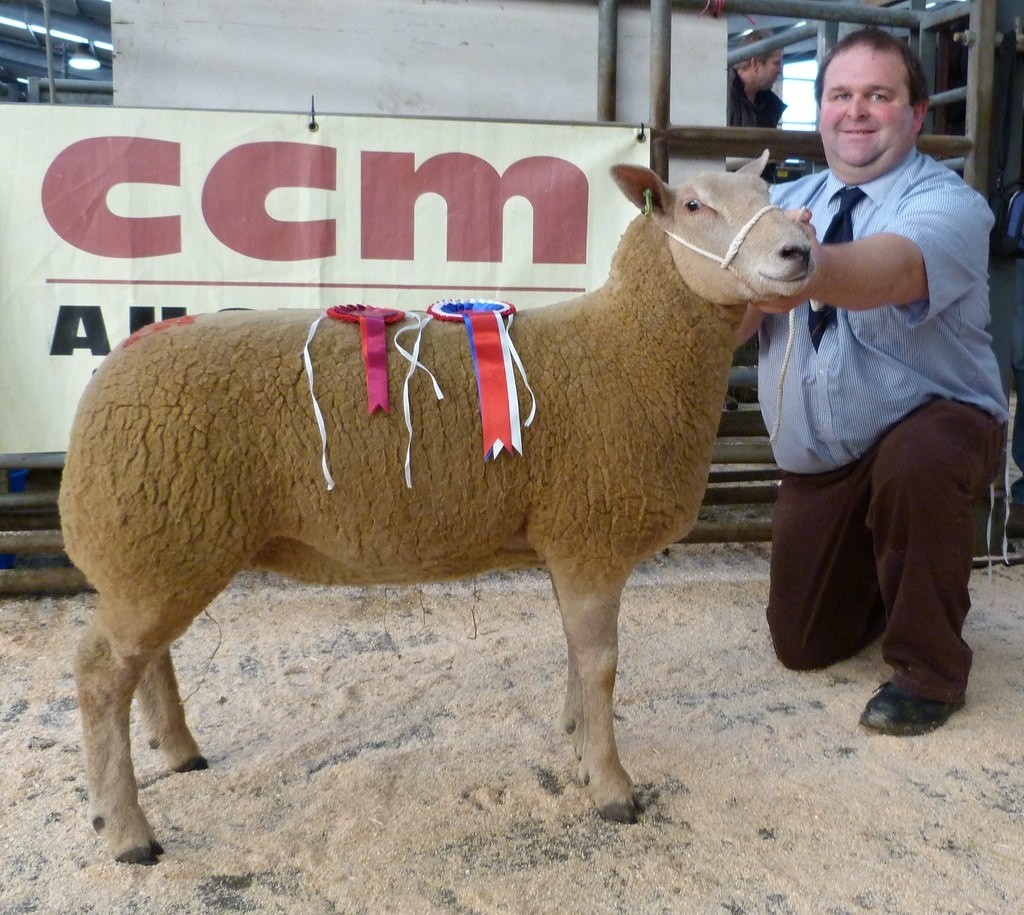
[807,186,867,353]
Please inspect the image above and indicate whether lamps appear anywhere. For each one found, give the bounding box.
[68,46,102,68]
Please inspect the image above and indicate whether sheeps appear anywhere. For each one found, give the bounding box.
[57,148,811,866]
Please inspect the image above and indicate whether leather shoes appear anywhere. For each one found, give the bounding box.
[859,680,965,735]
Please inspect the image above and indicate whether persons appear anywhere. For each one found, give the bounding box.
[723,30,788,411]
[733,30,1009,738]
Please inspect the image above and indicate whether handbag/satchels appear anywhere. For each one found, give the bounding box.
[988,180,1024,259]
